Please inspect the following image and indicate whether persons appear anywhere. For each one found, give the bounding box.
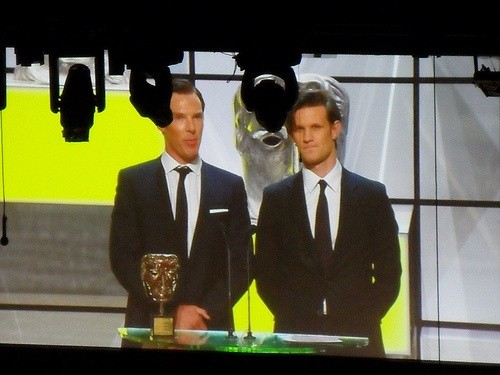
[108,77,255,332]
[254,92,403,346]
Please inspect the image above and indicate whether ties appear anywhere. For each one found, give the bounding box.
[316,178,333,253]
[173,166,193,258]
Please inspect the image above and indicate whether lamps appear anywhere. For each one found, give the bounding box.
[108,50,185,128]
[48,50,105,143]
[232,51,302,134]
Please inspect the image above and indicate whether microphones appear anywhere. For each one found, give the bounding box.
[243,225,257,339]
[218,222,237,339]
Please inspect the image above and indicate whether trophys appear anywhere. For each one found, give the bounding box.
[141,253,180,339]
